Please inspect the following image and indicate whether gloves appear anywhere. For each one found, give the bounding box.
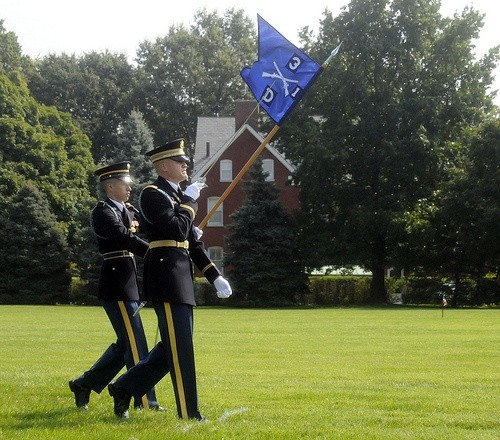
[183,180,205,200]
[195,227,203,240]
[213,276,232,298]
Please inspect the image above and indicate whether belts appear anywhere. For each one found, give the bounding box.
[149,239,189,250]
[103,250,133,261]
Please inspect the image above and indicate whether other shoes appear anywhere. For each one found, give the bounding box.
[69,374,91,410]
[108,382,129,418]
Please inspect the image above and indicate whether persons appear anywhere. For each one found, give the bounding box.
[68,161,167,412]
[109,139,232,420]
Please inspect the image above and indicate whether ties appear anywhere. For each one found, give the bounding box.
[122,206,130,227]
[178,186,183,198]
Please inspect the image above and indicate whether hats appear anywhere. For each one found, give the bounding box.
[94,161,132,183]
[146,138,192,167]
